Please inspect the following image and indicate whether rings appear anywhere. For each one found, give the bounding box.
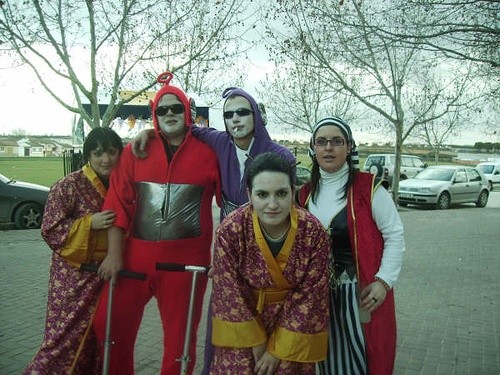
[372,297,377,303]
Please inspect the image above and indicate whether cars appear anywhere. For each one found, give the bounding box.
[398,165,492,210]
[475,161,500,191]
[296,166,313,186]
[0,174,50,229]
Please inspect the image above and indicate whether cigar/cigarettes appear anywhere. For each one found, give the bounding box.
[233,127,238,133]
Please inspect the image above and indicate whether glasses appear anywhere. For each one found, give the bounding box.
[156,103,185,117]
[223,108,253,119]
[314,138,344,146]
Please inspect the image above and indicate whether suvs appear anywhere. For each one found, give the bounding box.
[363,153,428,189]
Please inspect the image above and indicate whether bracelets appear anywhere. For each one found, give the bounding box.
[376,277,390,291]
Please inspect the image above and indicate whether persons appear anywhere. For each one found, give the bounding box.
[93,86,222,375]
[22,127,125,375]
[131,86,296,375]
[212,152,330,375]
[294,116,406,375]
